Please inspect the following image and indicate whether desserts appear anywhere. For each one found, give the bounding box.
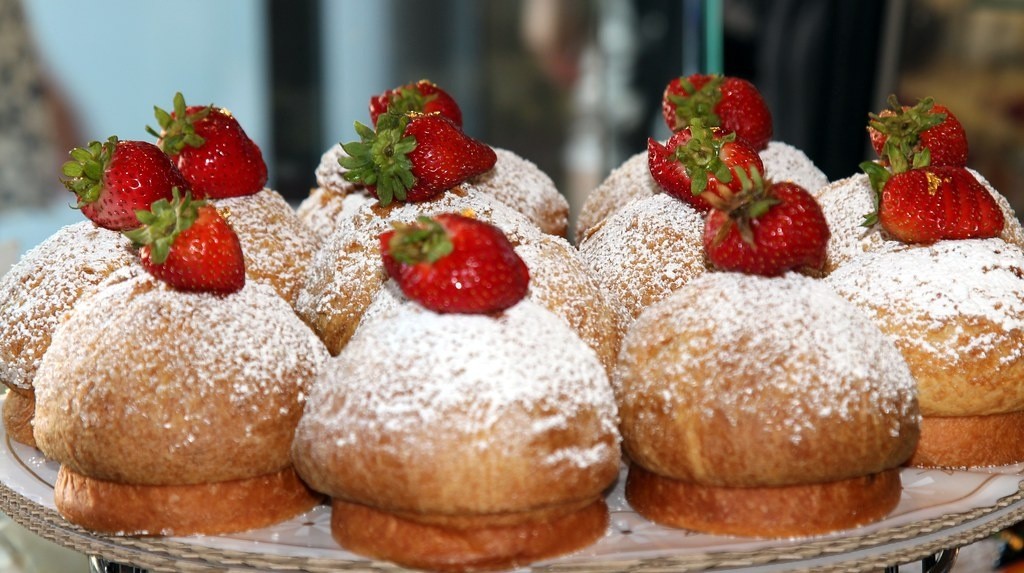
[0,69,1024,573]
